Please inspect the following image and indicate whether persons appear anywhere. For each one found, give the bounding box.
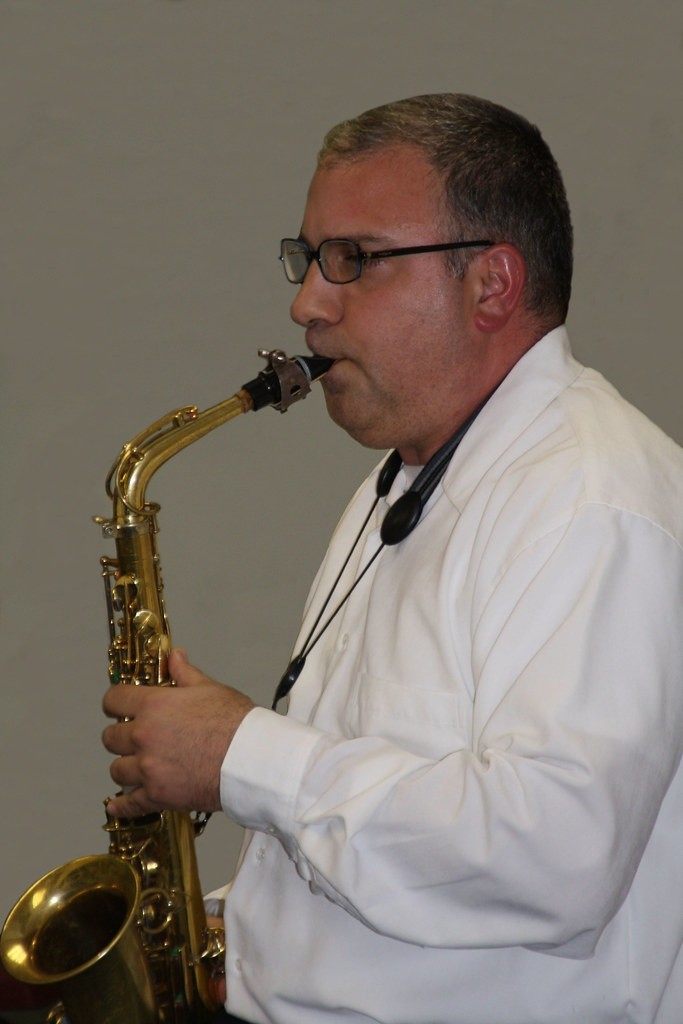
[101,92,683,1023]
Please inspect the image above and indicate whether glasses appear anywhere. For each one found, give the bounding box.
[278,238,493,284]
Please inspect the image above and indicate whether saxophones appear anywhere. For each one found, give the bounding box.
[1,348,341,1024]
